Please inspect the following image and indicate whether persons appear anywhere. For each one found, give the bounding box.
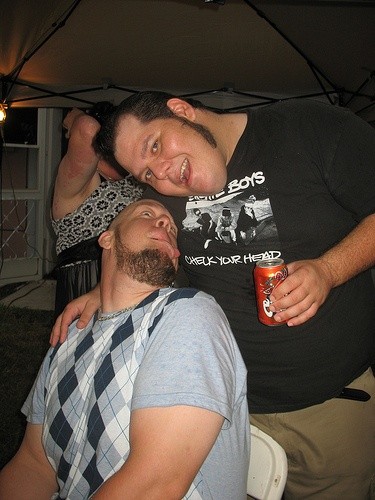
[0,200,250,500]
[91,90,375,500]
[50,100,146,321]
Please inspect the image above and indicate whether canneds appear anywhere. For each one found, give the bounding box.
[253,258,291,326]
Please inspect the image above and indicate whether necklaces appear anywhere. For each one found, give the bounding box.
[97,302,141,321]
[97,167,118,181]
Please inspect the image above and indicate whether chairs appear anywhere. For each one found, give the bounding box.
[247,424,289,500]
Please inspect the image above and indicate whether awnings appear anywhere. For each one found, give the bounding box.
[0,0,374,123]
